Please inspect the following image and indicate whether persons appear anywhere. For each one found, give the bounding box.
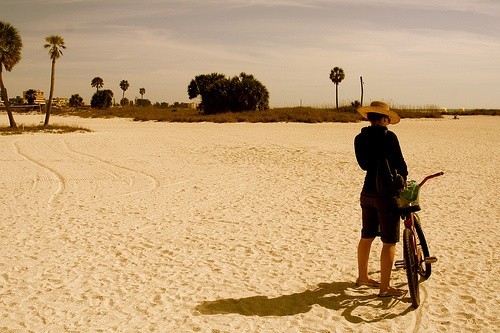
[353,102,408,298]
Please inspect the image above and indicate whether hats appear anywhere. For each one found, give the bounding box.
[357,101,400,124]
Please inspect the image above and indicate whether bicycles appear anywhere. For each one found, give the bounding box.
[394,172,443,308]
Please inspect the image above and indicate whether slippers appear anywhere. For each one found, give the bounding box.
[355,279,381,286]
[378,288,406,297]
